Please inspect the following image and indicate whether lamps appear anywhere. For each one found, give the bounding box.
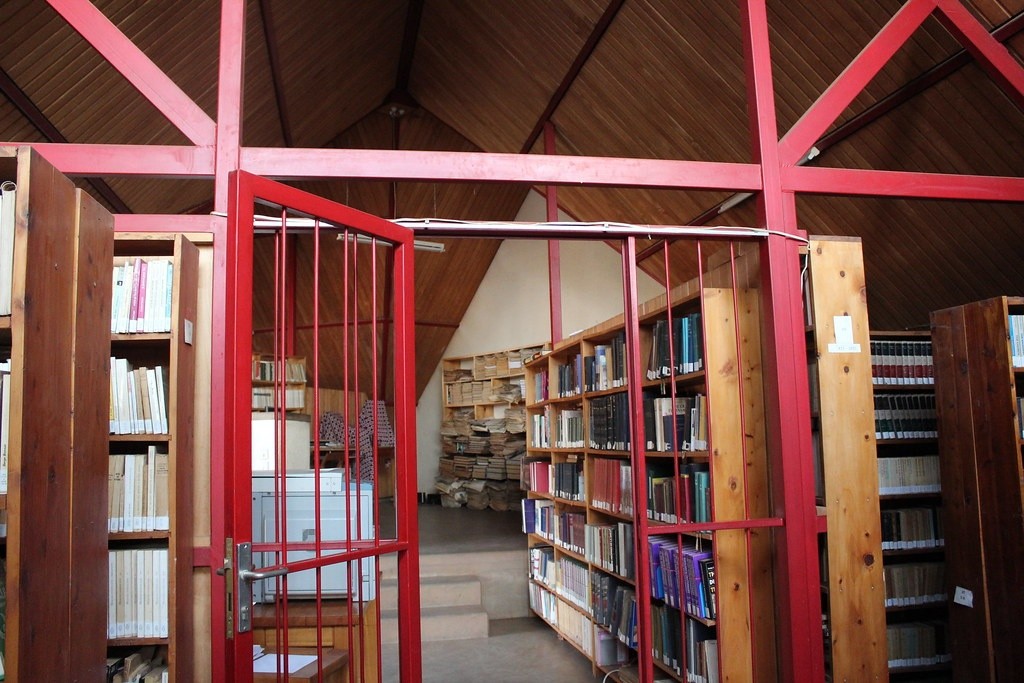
[717,147,820,214]
[337,179,446,251]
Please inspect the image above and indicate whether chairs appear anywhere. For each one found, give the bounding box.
[320,453,344,468]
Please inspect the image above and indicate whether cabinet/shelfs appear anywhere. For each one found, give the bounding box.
[109,230,200,683]
[0,144,106,683]
[252,352,308,414]
[803,325,951,676]
[442,342,544,481]
[525,252,778,683]
[798,235,889,683]
[929,296,1024,683]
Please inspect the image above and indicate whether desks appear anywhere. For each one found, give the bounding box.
[309,447,395,502]
[253,599,378,683]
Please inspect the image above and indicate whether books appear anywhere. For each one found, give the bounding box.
[531,405,549,449]
[1008,314,1024,439]
[558,354,582,398]
[589,393,631,452]
[592,457,634,519]
[648,464,712,534]
[520,455,585,500]
[584,332,626,392]
[651,603,718,683]
[648,536,716,619]
[251,360,307,409]
[869,342,947,673]
[435,348,530,493]
[108,259,171,639]
[646,314,703,380]
[528,544,590,653]
[584,522,634,578]
[591,571,638,648]
[521,498,587,555]
[534,371,549,403]
[555,404,584,448]
[644,393,708,452]
